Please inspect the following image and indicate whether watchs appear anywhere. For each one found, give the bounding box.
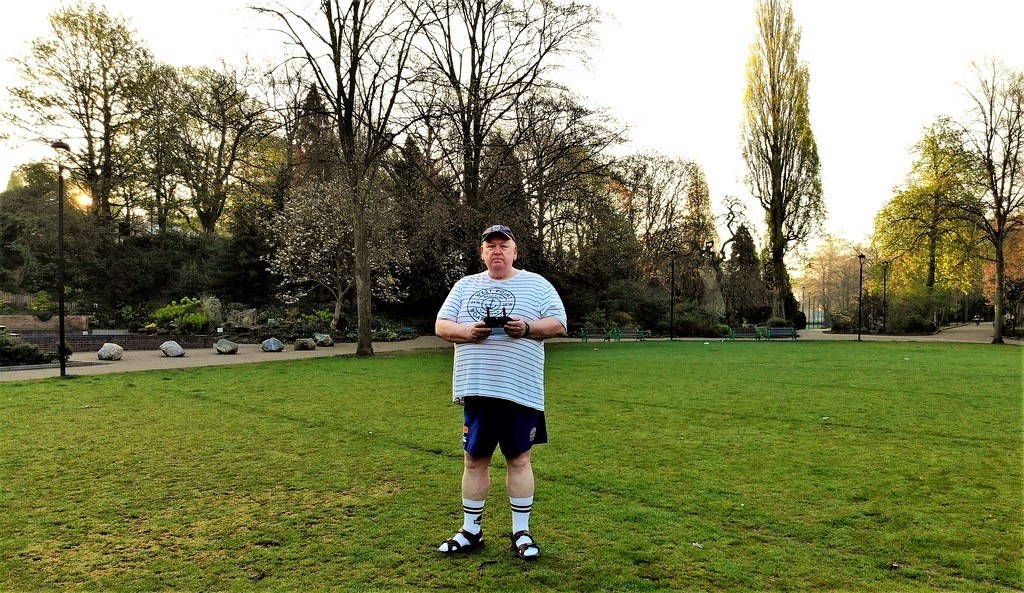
[523,321,530,337]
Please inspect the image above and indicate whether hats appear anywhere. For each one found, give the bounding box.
[481,224,516,243]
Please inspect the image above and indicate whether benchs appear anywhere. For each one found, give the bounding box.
[762,327,800,340]
[580,327,614,343]
[728,327,761,341]
[613,327,643,343]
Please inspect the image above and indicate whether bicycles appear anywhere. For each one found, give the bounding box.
[972,318,981,325]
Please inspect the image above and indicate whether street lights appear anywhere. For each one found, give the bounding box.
[669,250,678,340]
[49,140,72,375]
[882,260,889,333]
[802,287,822,328]
[858,253,864,342]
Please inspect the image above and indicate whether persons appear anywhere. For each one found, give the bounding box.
[974,313,981,325]
[436,225,567,558]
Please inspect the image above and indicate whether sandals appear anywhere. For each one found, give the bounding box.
[436,529,485,554]
[510,529,541,560]
